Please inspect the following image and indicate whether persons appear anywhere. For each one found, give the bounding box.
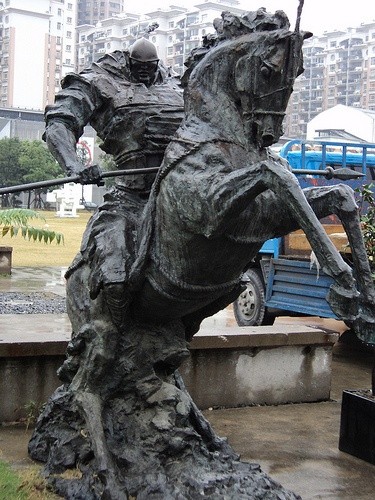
[42,36,190,376]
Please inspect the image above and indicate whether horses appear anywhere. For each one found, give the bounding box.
[68,3,375,499]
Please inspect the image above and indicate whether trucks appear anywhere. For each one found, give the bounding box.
[231,140,375,326]
